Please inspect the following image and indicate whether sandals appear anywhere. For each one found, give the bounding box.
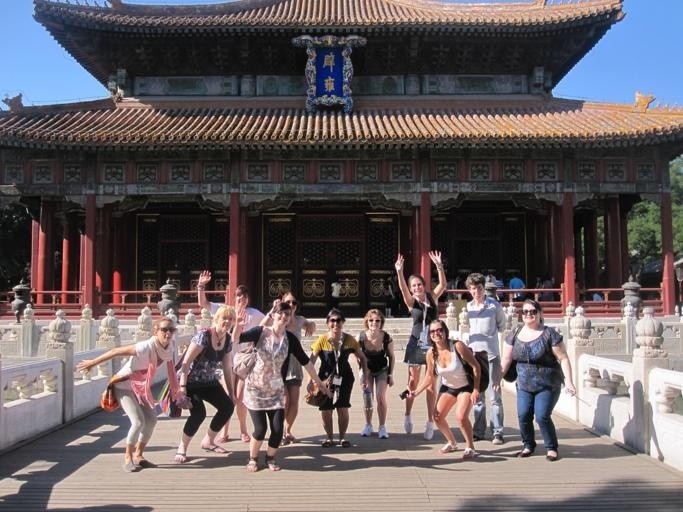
[546,449,558,461]
[264,455,280,471]
[246,456,259,472]
[441,441,457,453]
[514,447,534,457]
[463,447,475,460]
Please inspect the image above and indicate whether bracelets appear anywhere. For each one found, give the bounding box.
[388,374,393,377]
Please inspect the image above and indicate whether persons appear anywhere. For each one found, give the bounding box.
[199,270,266,444]
[258,290,315,444]
[509,272,526,300]
[494,295,577,462]
[541,273,557,300]
[174,307,238,464]
[231,302,333,472]
[490,272,501,302]
[76,318,181,474]
[395,249,447,442]
[383,274,394,320]
[309,310,368,448]
[535,275,544,289]
[328,276,342,307]
[355,308,395,441]
[465,273,507,445]
[405,320,482,458]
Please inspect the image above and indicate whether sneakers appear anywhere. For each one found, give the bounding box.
[404,416,413,434]
[361,423,373,436]
[423,422,434,441]
[378,425,389,439]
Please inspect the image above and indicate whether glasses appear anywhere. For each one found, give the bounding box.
[286,299,297,306]
[330,318,341,323]
[522,309,537,315]
[160,327,176,333]
[368,319,380,323]
[430,328,442,333]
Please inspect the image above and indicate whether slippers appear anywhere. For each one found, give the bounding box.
[472,435,478,440]
[121,461,138,472]
[133,458,156,468]
[201,445,228,454]
[175,452,187,462]
[491,435,504,445]
[322,440,333,447]
[339,439,350,448]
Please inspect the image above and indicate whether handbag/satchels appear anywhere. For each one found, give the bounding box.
[232,326,266,380]
[304,334,348,406]
[454,340,489,391]
[157,327,210,418]
[101,358,162,413]
[418,292,431,348]
[504,325,523,382]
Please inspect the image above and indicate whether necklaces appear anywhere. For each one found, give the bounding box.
[214,330,225,346]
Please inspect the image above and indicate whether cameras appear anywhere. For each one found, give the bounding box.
[398,390,409,400]
